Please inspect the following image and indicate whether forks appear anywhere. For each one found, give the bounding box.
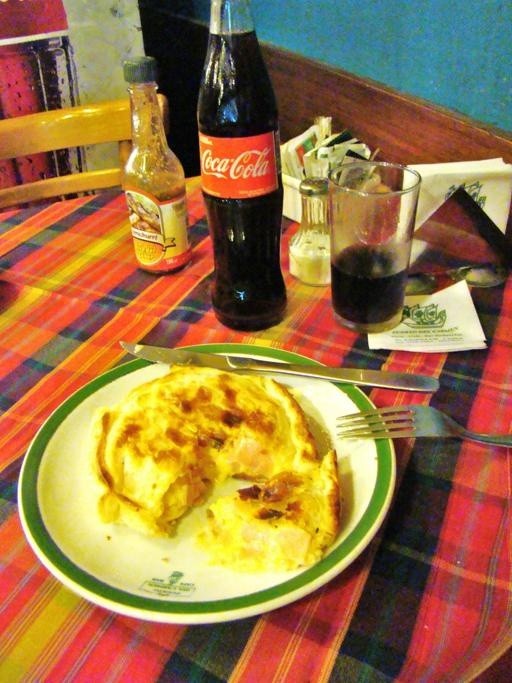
[333,404,512,450]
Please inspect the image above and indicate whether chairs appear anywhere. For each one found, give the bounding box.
[0,93,172,208]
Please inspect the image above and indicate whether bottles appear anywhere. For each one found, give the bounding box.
[291,175,333,287]
[120,55,192,275]
[196,0,287,333]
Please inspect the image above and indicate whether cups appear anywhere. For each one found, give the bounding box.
[328,160,420,329]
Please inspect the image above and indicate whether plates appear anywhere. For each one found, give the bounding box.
[17,341,397,624]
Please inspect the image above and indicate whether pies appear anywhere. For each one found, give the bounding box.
[91,365,340,574]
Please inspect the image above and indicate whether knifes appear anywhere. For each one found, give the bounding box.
[118,338,439,394]
